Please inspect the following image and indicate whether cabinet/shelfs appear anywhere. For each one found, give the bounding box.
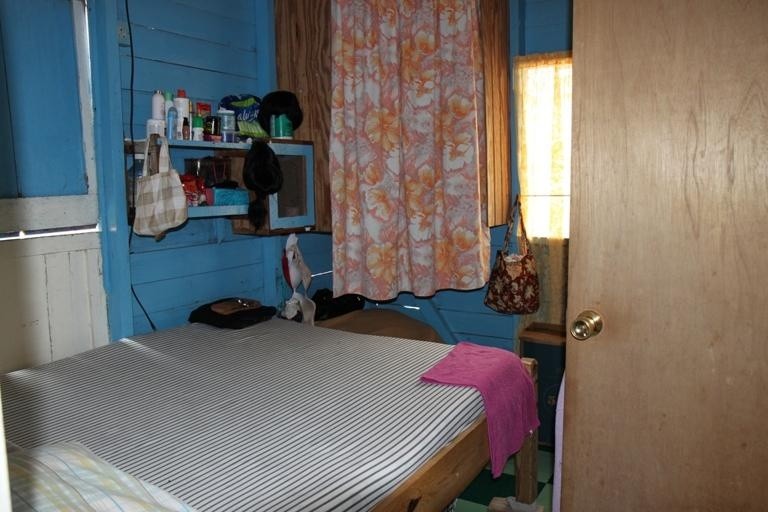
[513,323,565,449]
[125,130,253,223]
[231,141,317,237]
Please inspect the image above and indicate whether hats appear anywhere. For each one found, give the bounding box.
[256,91,303,136]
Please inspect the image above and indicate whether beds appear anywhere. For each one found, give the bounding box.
[0,306,540,512]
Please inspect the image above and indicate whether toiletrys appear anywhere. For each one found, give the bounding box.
[146,86,238,143]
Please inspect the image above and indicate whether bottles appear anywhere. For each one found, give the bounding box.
[127,154,151,214]
[150,88,236,142]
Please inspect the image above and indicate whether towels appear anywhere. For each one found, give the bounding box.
[419,338,542,477]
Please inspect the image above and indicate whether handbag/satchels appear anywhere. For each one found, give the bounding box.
[133,133,188,237]
[484,193,540,315]
[189,297,277,329]
